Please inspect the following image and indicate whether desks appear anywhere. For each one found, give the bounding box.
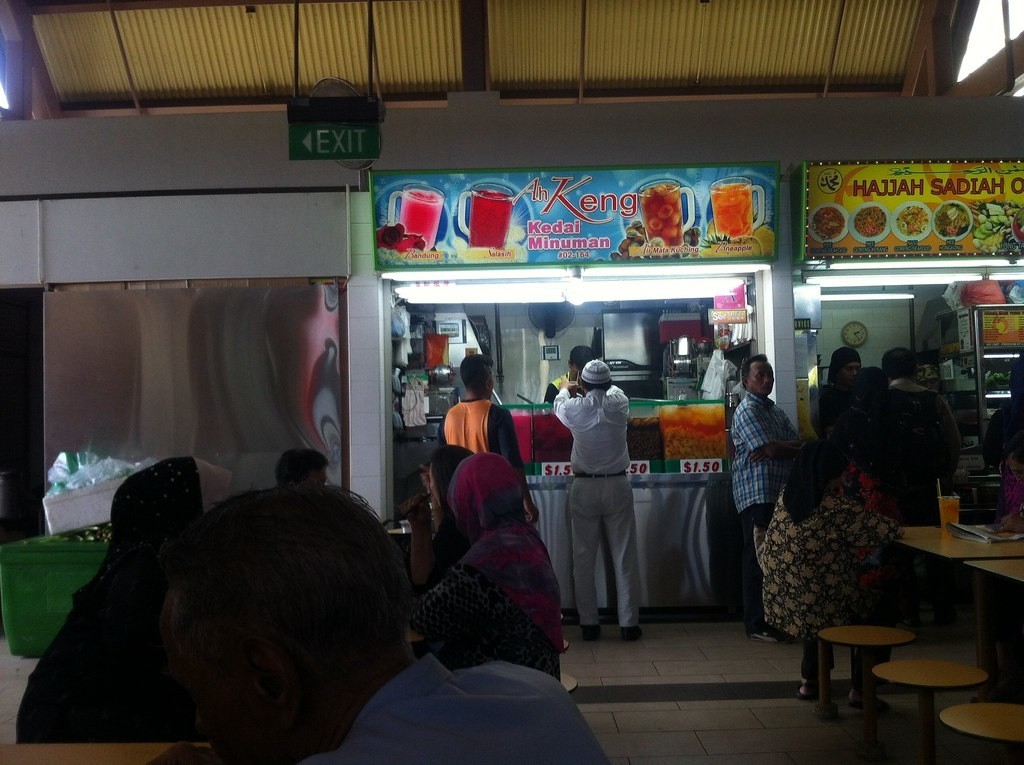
[0,742,212,765]
[387,528,411,541]
[962,558,1024,702]
[892,525,1024,559]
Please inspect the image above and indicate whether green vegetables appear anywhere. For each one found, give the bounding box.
[985,372,1011,392]
[939,203,969,236]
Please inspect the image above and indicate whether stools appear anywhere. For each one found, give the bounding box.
[817,625,918,763]
[938,703,1024,765]
[873,658,990,765]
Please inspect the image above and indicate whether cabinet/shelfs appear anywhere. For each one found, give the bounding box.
[933,303,1024,475]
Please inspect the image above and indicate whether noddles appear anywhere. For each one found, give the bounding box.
[933,203,971,238]
[896,205,929,236]
[812,207,844,239]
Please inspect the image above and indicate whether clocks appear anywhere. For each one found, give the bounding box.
[841,320,868,349]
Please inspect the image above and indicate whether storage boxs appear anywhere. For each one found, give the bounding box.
[410,324,424,339]
[666,377,698,401]
[659,399,728,473]
[407,339,427,369]
[963,444,984,454]
[0,536,111,658]
[658,313,702,345]
[532,403,573,475]
[42,462,140,535]
[962,435,979,448]
[958,454,985,471]
[494,404,535,475]
[406,374,430,391]
[405,370,425,375]
[626,401,664,473]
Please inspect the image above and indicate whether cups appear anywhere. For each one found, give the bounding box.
[386,182,446,253]
[637,178,696,246]
[459,182,516,250]
[709,176,765,240]
[399,519,412,534]
[937,496,960,535]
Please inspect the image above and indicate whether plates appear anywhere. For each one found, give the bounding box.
[932,199,973,242]
[808,203,849,244]
[1012,219,1023,242]
[890,200,932,242]
[847,202,891,244]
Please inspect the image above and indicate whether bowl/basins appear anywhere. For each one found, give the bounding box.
[1013,207,1024,240]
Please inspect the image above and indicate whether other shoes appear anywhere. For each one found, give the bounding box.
[621,624,642,641]
[581,624,601,641]
[934,605,957,626]
[902,614,922,627]
[749,627,793,644]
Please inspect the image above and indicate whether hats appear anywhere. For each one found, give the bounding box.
[581,359,611,384]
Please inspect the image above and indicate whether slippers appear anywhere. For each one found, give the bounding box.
[797,683,819,701]
[848,697,890,712]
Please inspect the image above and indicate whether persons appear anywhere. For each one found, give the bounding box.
[436,354,539,524]
[983,355,1024,526]
[160,484,613,765]
[855,346,961,526]
[818,347,862,436]
[543,345,598,403]
[273,447,328,484]
[553,359,642,642]
[731,353,804,644]
[403,450,562,682]
[754,442,905,713]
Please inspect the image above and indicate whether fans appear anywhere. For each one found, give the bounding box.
[528,302,575,338]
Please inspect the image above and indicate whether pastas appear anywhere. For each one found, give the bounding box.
[854,207,887,237]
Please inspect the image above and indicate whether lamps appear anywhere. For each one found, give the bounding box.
[825,256,1024,269]
[581,263,771,278]
[821,289,916,301]
[801,266,987,288]
[381,267,577,280]
[986,267,1024,280]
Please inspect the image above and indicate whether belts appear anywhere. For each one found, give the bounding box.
[575,470,626,478]
